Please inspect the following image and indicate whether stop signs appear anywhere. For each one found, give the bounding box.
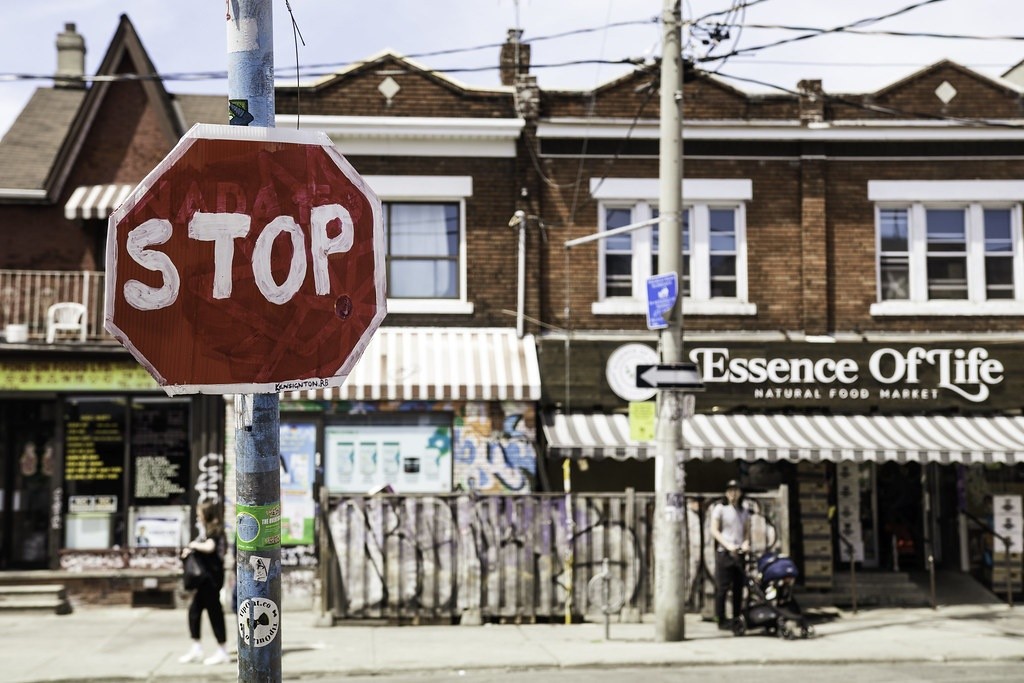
[104,123,387,396]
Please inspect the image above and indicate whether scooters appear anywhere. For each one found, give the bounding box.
[746,547,809,639]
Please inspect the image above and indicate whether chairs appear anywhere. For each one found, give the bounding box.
[45,302,89,345]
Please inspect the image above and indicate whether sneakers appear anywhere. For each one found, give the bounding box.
[203,648,231,666]
[178,643,203,662]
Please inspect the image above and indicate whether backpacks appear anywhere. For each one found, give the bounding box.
[178,545,219,590]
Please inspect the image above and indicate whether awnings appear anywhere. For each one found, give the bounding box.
[279,327,541,401]
[539,410,1024,466]
[64,184,136,220]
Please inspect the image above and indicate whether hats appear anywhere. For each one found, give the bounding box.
[723,479,742,491]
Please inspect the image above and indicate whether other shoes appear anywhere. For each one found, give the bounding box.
[732,617,742,630]
[718,616,730,628]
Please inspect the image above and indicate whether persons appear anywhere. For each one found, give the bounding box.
[137,526,149,546]
[178,502,231,666]
[710,479,751,630]
